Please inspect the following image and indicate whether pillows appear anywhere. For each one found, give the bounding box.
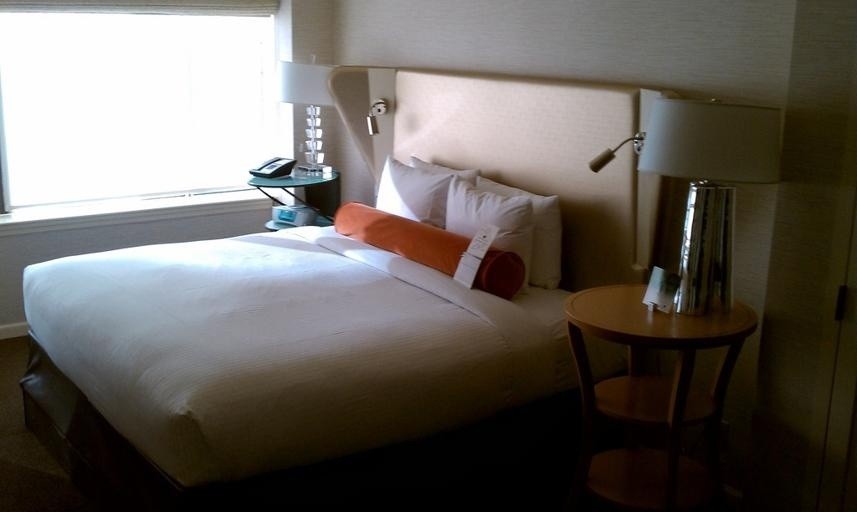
[333,154,563,303]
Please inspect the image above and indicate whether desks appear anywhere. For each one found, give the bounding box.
[247,166,342,231]
[560,280,762,512]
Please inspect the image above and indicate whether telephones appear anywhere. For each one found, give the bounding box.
[249,157,296,177]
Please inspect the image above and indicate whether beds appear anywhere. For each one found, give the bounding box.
[20,63,679,512]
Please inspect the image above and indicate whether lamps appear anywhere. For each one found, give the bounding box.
[272,52,343,177]
[631,96,784,314]
